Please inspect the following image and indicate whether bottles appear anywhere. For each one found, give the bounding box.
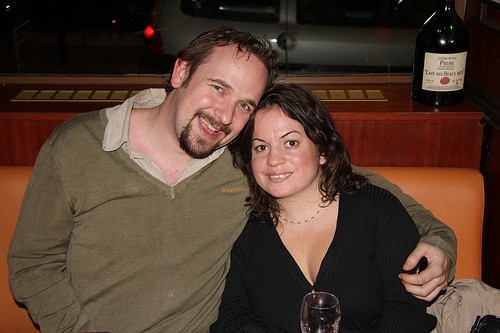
[411,0,470,106]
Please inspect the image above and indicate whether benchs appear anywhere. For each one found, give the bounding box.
[0,165,485,333]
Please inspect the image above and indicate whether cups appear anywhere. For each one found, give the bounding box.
[300,292,342,333]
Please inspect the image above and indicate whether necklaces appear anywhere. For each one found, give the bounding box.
[265,195,328,226]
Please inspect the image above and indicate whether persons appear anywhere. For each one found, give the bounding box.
[12,25,461,333]
[208,81,439,332]
